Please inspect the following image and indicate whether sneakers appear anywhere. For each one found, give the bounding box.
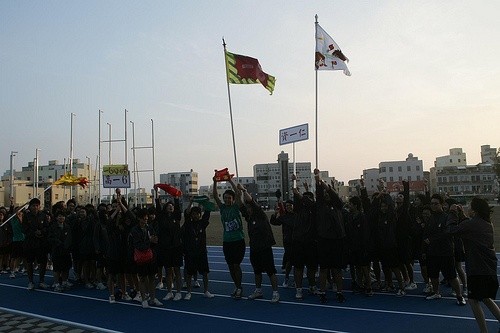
[282,266,469,306]
[0,263,215,309]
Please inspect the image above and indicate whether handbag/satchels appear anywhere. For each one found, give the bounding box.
[133,247,154,264]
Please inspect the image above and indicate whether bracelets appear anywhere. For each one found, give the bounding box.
[243,189,247,191]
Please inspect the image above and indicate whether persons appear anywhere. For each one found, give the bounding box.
[0,168,500,333]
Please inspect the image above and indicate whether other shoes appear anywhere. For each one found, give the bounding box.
[271,293,280,303]
[230,288,243,299]
[248,291,263,299]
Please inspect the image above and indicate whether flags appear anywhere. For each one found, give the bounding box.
[225,48,276,95]
[315,23,351,76]
[52,172,90,189]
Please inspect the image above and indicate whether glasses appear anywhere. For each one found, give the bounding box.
[68,203,75,208]
[285,205,293,207]
[430,202,440,206]
